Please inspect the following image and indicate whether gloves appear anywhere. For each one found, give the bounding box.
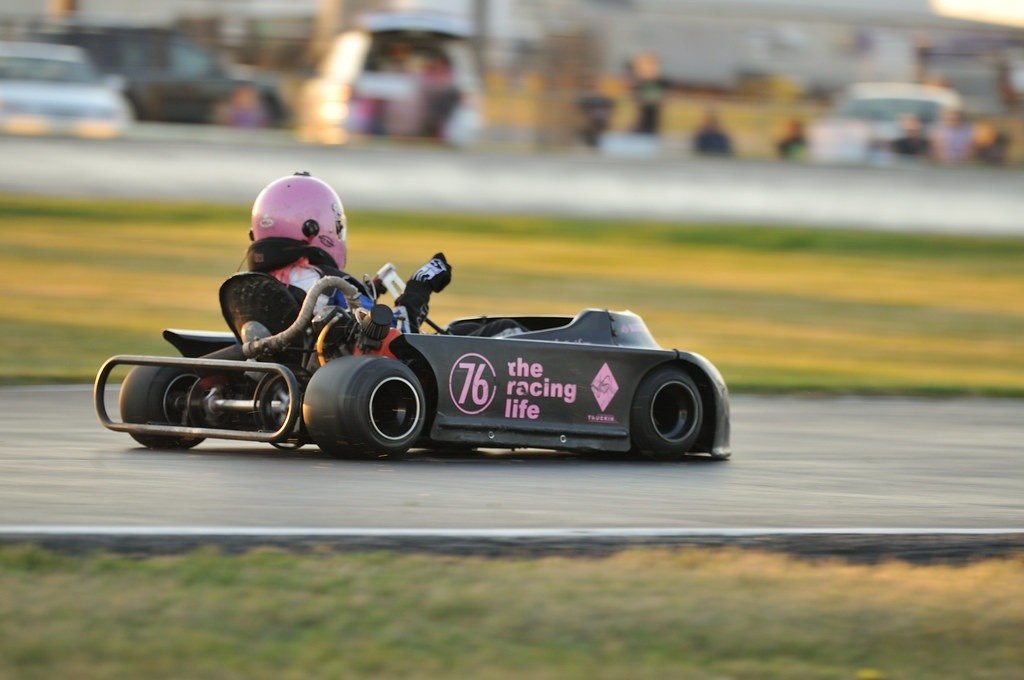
[394,253,452,311]
[362,279,386,299]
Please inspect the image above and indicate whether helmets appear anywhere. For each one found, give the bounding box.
[250,172,346,269]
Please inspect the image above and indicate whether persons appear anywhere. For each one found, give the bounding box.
[213,51,1021,163]
[247,172,530,359]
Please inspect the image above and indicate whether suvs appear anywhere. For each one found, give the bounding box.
[297,16,482,147]
[50,22,293,129]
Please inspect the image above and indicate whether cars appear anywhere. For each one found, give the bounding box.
[805,83,968,172]
[0,41,137,133]
